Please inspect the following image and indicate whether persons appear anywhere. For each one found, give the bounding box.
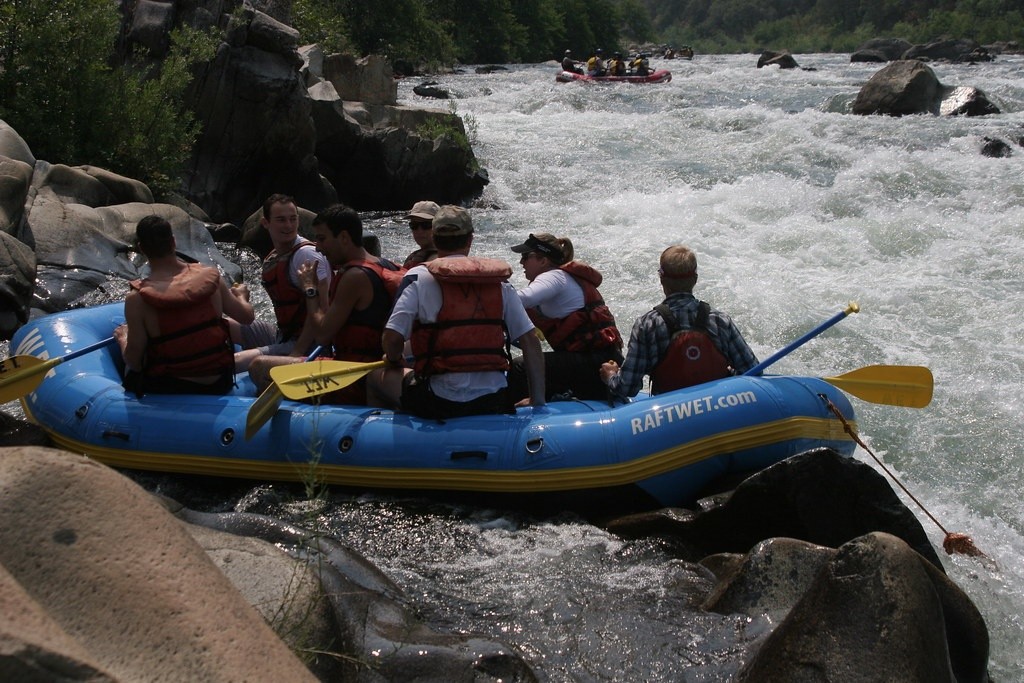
[587,48,649,76]
[247,204,409,406]
[507,232,623,401]
[562,49,584,74]
[366,203,546,421]
[403,200,441,267]
[113,214,255,395]
[598,244,764,398]
[224,193,328,375]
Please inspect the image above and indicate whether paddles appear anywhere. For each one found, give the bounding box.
[269,327,544,401]
[609,359,934,409]
[0,281,239,406]
[742,300,859,376]
[245,344,326,441]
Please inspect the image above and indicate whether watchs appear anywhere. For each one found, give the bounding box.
[303,287,320,297]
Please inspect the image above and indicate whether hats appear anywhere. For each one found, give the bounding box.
[510,233,564,260]
[431,204,473,236]
[402,201,442,219]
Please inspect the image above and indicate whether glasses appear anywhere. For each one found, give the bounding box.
[521,251,545,261]
[408,221,432,231]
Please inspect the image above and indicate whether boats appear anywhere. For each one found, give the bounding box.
[8,300,859,520]
[556,69,674,84]
